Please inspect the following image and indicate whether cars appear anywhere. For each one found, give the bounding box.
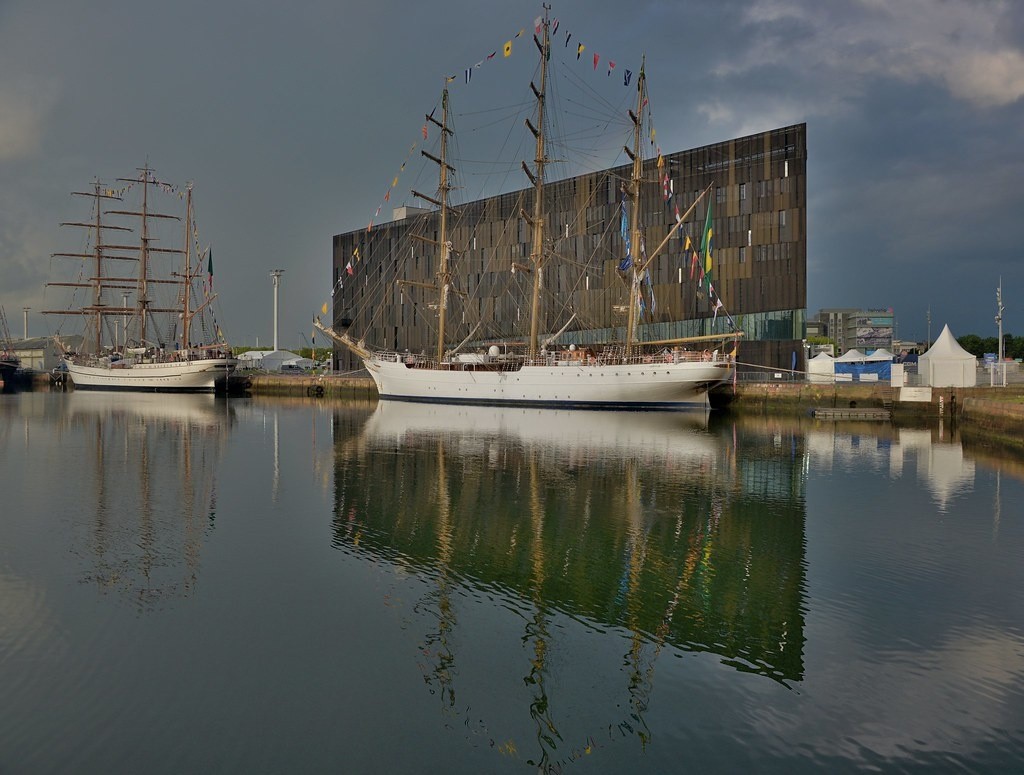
[982,359,1020,373]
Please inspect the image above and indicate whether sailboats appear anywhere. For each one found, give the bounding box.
[39,150,243,392]
[307,2,746,413]
[354,401,736,774]
[59,391,241,619]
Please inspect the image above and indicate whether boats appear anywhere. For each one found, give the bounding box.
[0,303,22,392]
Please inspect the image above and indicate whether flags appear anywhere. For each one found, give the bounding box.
[593,51,600,71]
[622,68,633,87]
[563,29,572,48]
[606,59,617,77]
[576,41,586,61]
[533,15,543,37]
[55,169,231,351]
[552,15,560,37]
[308,121,430,377]
[445,24,525,85]
[641,72,724,327]
